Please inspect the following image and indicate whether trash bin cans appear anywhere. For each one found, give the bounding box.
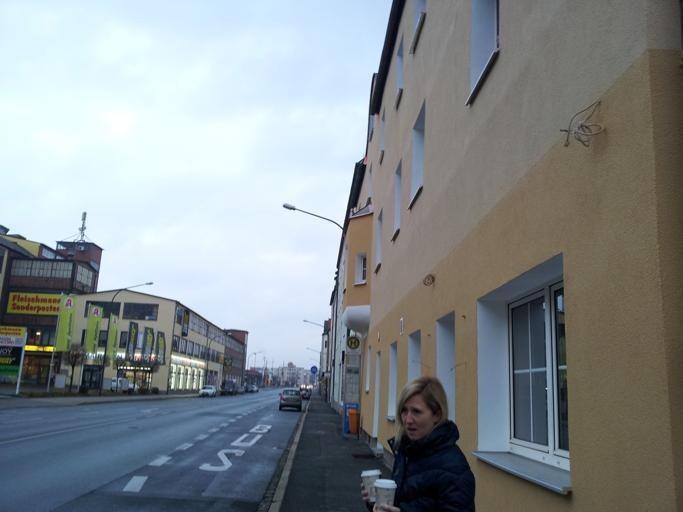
[348,408,359,434]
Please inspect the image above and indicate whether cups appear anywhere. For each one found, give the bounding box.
[361,469,396,510]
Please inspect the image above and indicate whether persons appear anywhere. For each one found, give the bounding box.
[360,375,476,512]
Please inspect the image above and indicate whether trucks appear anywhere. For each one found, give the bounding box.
[219,379,237,395]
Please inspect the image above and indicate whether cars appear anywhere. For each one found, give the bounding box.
[237,384,259,393]
[300,388,313,401]
[198,384,217,398]
[109,376,141,394]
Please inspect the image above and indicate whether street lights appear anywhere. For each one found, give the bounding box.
[98,281,153,395]
[246,351,262,371]
[204,331,234,385]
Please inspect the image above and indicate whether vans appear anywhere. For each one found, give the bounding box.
[277,387,302,412]
